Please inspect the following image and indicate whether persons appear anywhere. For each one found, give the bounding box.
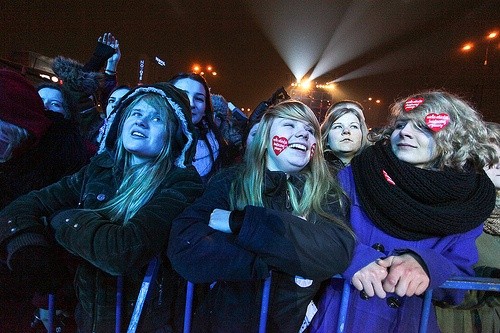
[167,98,355,333]
[0,33,269,333]
[306,91,500,333]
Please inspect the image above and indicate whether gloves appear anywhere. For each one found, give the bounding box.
[265,86,292,106]
